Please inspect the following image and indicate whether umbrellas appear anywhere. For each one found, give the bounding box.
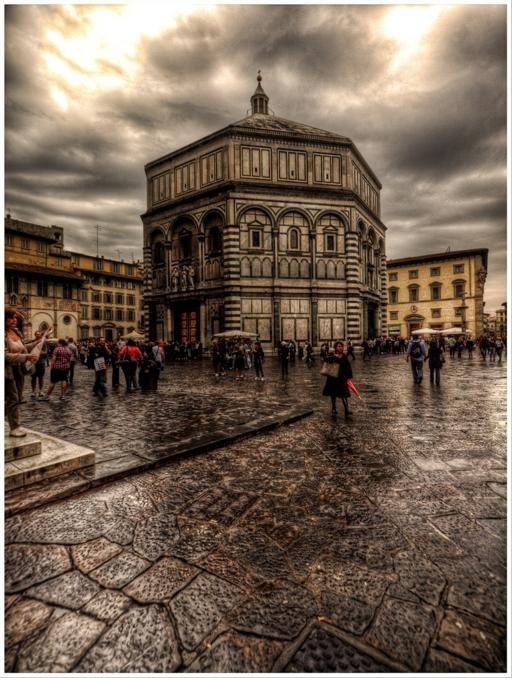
[346,379,360,398]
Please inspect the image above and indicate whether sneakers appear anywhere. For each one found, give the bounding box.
[214,371,246,381]
[254,377,266,381]
[17,392,65,404]
[412,382,440,388]
[94,388,104,401]
[9,427,27,437]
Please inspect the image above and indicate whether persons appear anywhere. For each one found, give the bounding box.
[320,342,353,416]
[28,332,266,402]
[278,335,504,385]
[5,306,42,438]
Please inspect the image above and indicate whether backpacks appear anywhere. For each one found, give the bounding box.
[410,340,423,358]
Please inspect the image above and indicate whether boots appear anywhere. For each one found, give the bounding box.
[281,374,289,382]
[126,375,138,392]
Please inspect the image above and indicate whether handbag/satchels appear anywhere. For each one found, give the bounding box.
[319,361,340,379]
[122,346,132,365]
[93,356,107,372]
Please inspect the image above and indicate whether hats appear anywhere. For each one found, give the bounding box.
[281,340,289,346]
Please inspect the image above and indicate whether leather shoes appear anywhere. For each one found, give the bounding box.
[331,407,354,416]
